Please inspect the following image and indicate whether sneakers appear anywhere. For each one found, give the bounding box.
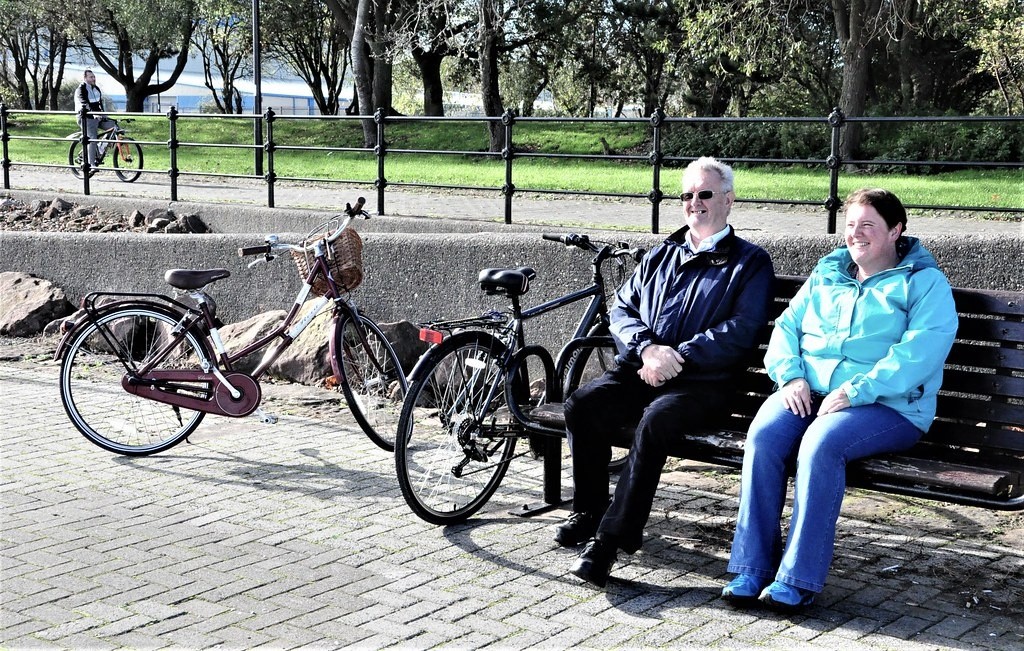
[554,493,614,546]
[720,569,771,600]
[758,577,818,606]
[569,536,618,588]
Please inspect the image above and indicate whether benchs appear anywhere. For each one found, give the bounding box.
[504,275,1023,519]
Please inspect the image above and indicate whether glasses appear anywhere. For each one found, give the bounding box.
[678,190,731,201]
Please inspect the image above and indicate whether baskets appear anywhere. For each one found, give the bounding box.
[289,227,362,296]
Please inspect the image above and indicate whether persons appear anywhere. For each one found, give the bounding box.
[74,70,118,171]
[552,156,777,587]
[720,187,960,611]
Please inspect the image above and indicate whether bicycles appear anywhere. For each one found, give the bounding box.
[394,236,647,526]
[54,197,414,456]
[66,116,143,182]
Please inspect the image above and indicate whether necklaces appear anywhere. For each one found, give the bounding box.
[857,270,860,281]
